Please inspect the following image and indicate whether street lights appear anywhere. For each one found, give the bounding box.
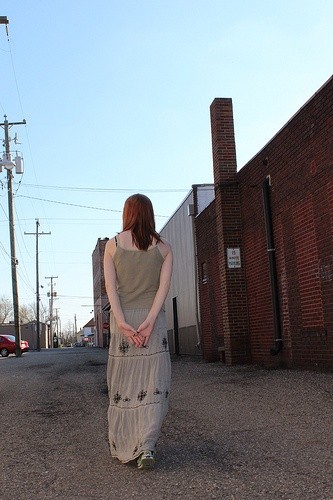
[45,276,59,349]
[0,114,27,358]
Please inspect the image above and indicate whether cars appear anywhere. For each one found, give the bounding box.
[75,342,82,348]
[0,335,29,358]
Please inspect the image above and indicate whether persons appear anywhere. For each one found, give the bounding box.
[103,193,173,469]
